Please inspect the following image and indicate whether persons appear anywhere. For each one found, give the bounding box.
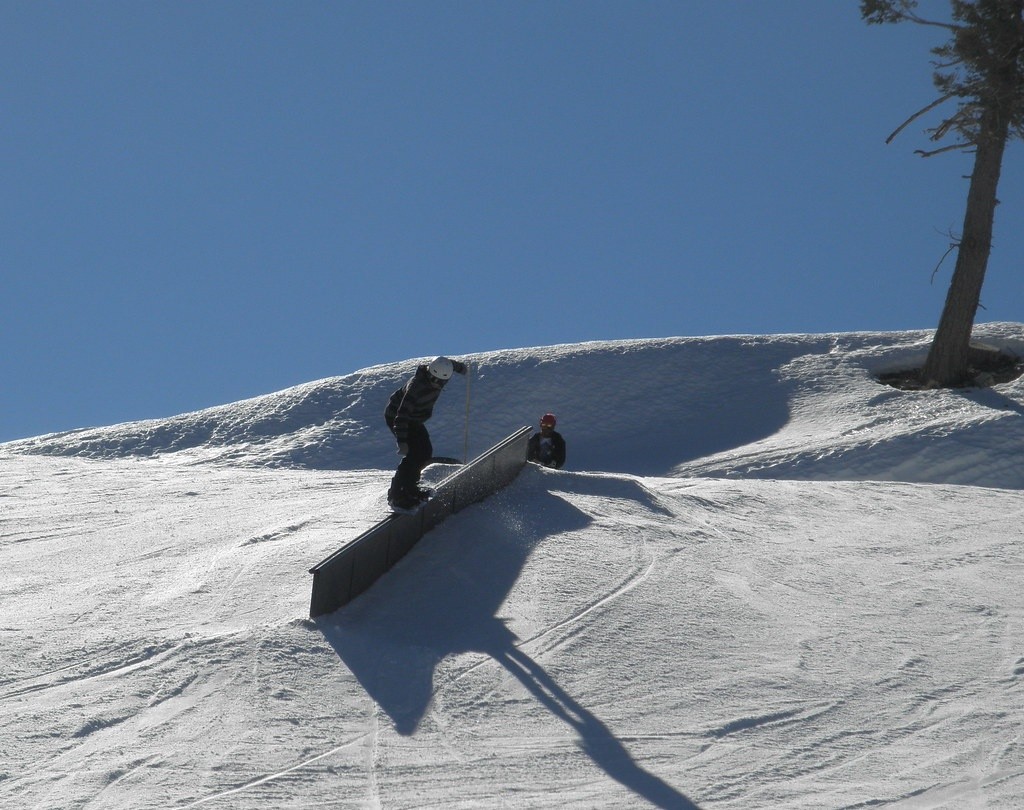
[384,357,468,507]
[529,414,566,469]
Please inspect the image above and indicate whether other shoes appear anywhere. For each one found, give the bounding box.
[387,489,420,509]
[402,483,434,499]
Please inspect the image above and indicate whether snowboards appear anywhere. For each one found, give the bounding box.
[384,486,438,516]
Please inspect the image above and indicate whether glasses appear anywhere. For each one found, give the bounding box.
[428,373,446,386]
[541,423,552,428]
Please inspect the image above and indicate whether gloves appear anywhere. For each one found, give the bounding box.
[460,363,467,375]
[396,442,409,456]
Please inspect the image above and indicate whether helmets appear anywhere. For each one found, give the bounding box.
[429,356,453,380]
[541,413,555,426]
